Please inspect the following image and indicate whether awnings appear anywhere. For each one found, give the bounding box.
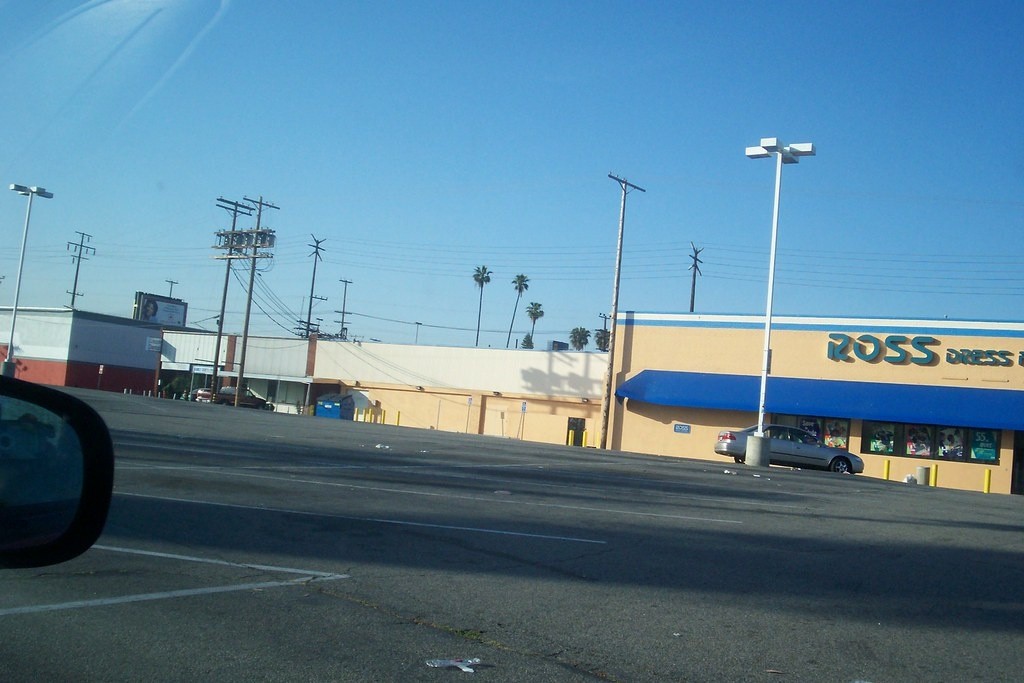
[615,370,1024,436]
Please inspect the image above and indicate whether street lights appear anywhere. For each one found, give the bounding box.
[744,138,818,467]
[2,183,54,378]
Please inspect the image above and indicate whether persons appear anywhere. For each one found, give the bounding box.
[141,299,159,322]
[800,418,819,437]
[940,430,963,455]
[825,422,847,446]
[908,425,930,454]
[875,429,894,451]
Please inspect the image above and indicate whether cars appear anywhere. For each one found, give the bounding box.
[715,422,866,474]
[188,389,216,401]
[217,386,265,408]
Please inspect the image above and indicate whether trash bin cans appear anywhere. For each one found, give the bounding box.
[315,392,355,420]
[916,466,930,485]
